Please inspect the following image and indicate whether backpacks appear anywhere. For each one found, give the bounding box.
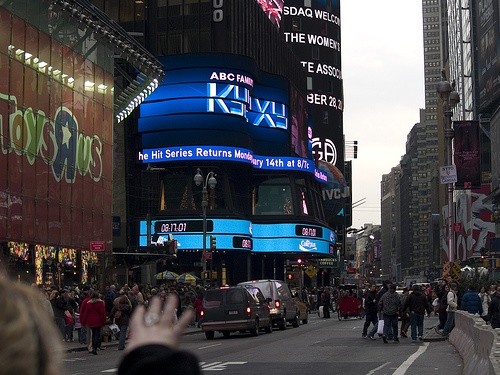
[442,291,455,304]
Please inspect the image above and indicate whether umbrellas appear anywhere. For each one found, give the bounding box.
[154,270,179,280]
[175,272,201,283]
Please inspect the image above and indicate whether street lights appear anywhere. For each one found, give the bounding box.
[194,167,218,272]
[354,233,375,261]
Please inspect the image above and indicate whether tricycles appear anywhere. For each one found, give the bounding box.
[337,283,365,321]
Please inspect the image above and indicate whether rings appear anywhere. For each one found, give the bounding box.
[144,312,160,325]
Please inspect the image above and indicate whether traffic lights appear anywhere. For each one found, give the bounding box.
[210,236,216,250]
[297,258,302,264]
[288,273,292,280]
[480,247,485,259]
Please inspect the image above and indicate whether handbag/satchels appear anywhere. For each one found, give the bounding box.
[377,319,384,336]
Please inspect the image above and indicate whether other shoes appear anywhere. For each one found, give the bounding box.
[362,326,449,344]
[63,338,125,354]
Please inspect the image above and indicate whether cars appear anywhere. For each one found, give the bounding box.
[292,290,309,324]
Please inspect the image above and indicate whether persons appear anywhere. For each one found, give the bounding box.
[377,283,403,344]
[0,266,204,375]
[290,275,500,343]
[38,281,227,356]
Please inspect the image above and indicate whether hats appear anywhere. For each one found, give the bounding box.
[402,288,410,294]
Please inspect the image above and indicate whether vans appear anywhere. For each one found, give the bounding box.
[238,278,300,330]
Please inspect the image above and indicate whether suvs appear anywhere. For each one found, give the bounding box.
[201,284,274,339]
